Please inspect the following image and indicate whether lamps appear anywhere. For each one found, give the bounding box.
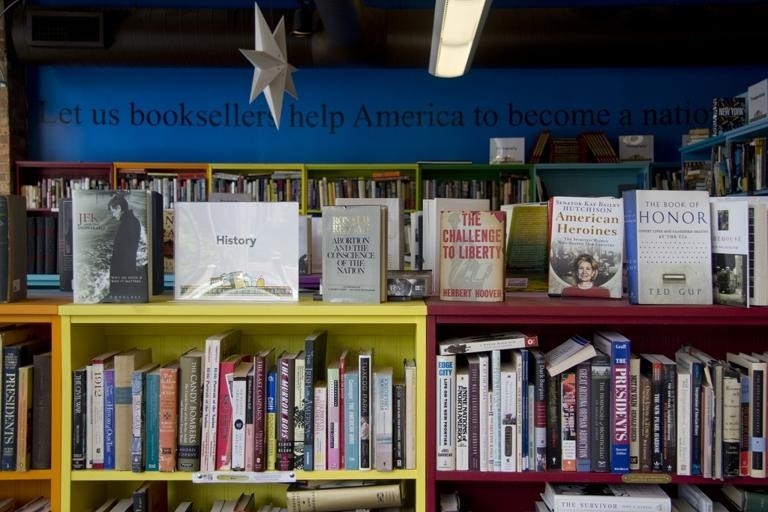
[427,0,492,79]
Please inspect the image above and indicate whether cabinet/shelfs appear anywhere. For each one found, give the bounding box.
[1,304,62,512]
[59,304,425,511]
[678,118,767,197]
[426,304,768,512]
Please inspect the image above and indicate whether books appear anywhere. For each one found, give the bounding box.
[1,76,767,512]
